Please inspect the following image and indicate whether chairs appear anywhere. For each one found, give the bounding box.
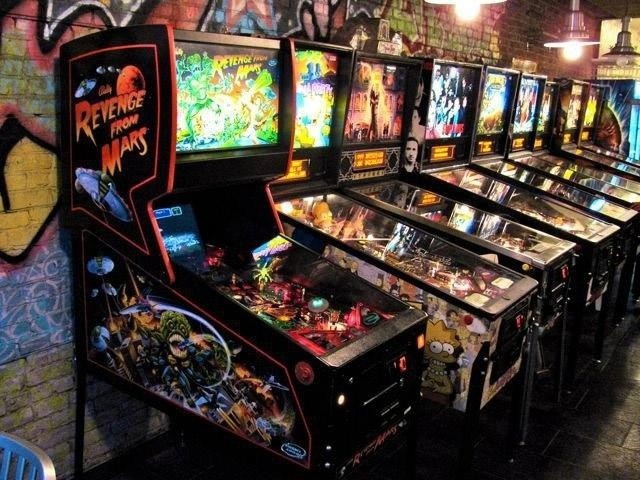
[1,429,57,479]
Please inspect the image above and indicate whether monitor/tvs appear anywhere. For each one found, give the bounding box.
[153,201,207,258]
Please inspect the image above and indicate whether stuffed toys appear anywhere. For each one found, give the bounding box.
[311,199,334,229]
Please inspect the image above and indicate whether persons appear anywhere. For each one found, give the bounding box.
[408,105,425,145]
[402,133,419,173]
[542,95,550,115]
[518,80,535,123]
[424,69,473,140]
[413,74,427,108]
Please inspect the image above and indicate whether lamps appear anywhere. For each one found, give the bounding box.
[541,1,603,63]
[602,1,640,66]
[426,0,511,22]
